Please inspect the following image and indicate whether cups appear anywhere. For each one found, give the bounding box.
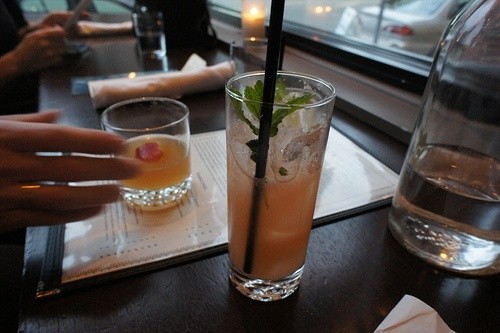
[132,11,166,56]
[224,70,336,301]
[229,38,282,76]
[100,96,194,210]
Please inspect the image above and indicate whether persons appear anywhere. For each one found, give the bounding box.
[0,0,91,80]
[0,109,147,231]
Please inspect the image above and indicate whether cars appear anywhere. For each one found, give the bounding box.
[357,0,466,57]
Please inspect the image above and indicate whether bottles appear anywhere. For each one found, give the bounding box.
[386,0,500,273]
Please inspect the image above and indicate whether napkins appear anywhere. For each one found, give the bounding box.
[373,294,457,333]
